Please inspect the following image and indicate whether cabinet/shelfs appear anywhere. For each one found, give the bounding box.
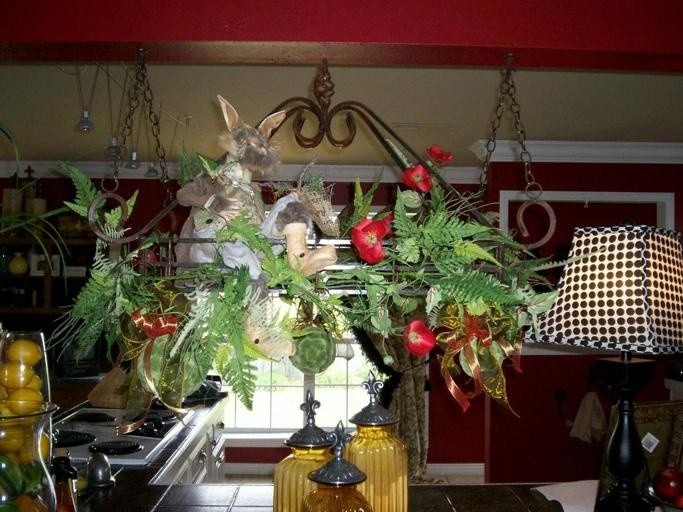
[1,174,183,385]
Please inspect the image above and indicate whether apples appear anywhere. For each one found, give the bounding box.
[652,464,683,510]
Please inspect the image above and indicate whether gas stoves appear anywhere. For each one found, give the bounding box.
[48,407,207,465]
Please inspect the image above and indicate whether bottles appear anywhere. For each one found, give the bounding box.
[0,330,78,512]
[9,253,28,274]
[0,254,8,272]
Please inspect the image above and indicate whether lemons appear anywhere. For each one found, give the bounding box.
[0,338,51,465]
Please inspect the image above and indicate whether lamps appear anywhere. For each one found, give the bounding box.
[344,372,411,512]
[71,62,163,178]
[299,425,375,511]
[521,223,683,511]
[271,388,336,511]
